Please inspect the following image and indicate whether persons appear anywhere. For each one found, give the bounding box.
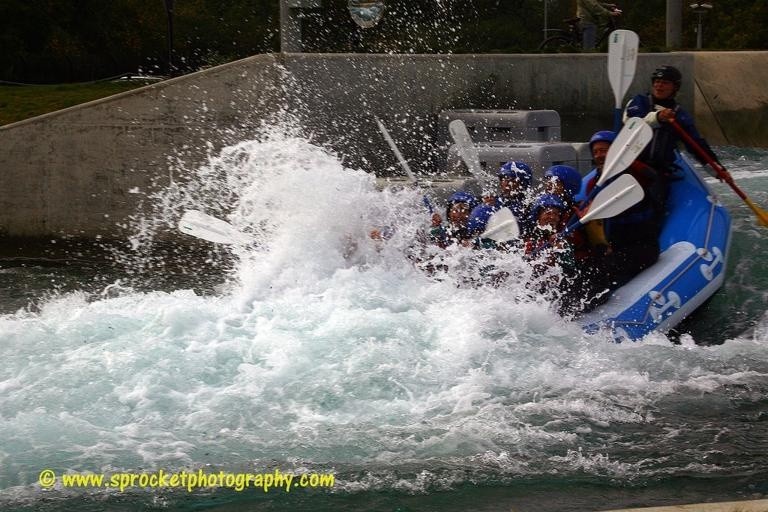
[370,130,617,309]
[622,65,732,274]
[576,1,622,54]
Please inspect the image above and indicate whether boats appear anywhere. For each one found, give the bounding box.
[560,144,732,355]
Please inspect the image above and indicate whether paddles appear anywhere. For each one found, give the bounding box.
[607,27,639,128]
[458,207,520,250]
[536,173,644,256]
[569,116,653,224]
[375,114,451,239]
[660,104,768,229]
[447,117,499,212]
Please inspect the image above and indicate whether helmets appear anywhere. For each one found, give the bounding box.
[587,130,617,157]
[447,190,499,238]
[498,160,532,179]
[650,64,682,89]
[532,194,564,224]
[542,165,582,196]
